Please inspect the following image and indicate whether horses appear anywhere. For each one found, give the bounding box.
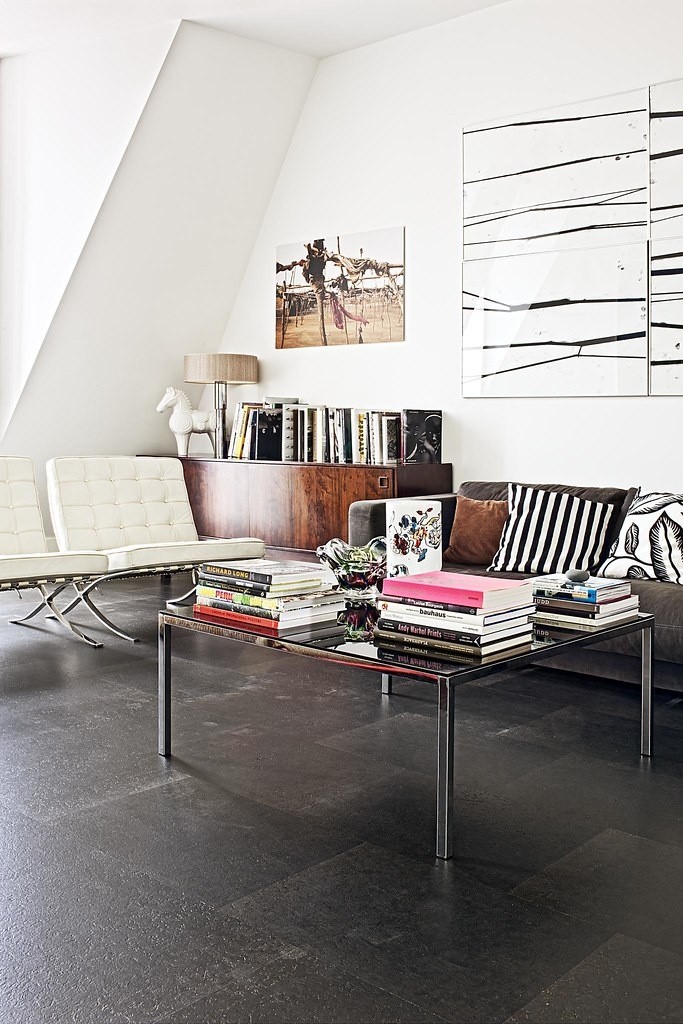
[157,384,230,456]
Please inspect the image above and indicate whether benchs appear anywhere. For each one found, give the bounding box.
[0,455,266,647]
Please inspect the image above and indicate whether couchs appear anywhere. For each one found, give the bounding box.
[347,480,683,696]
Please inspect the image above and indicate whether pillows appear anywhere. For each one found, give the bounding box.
[596,491,682,585]
[485,483,615,574]
[444,494,510,564]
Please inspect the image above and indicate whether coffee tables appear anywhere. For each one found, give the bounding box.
[155,564,655,859]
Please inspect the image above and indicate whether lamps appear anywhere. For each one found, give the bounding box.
[185,353,261,460]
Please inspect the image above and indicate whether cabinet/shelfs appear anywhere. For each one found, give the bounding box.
[134,452,456,562]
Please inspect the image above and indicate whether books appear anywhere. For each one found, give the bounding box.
[529,615,638,645]
[523,574,640,626]
[228,396,442,465]
[373,638,534,674]
[374,571,538,655]
[194,612,347,648]
[192,560,347,629]
